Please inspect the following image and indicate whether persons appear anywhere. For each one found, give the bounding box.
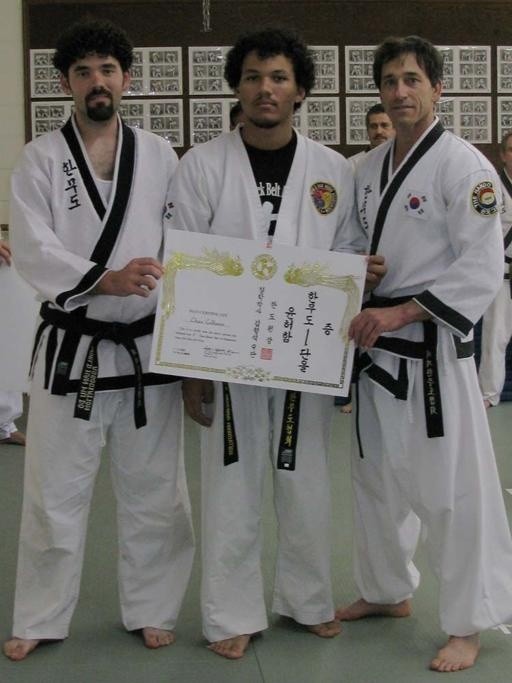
[3,17,200,662]
[477,132,512,408]
[162,25,354,662]
[334,103,395,415]
[330,35,512,673]
[0,240,27,446]
[229,100,245,132]
